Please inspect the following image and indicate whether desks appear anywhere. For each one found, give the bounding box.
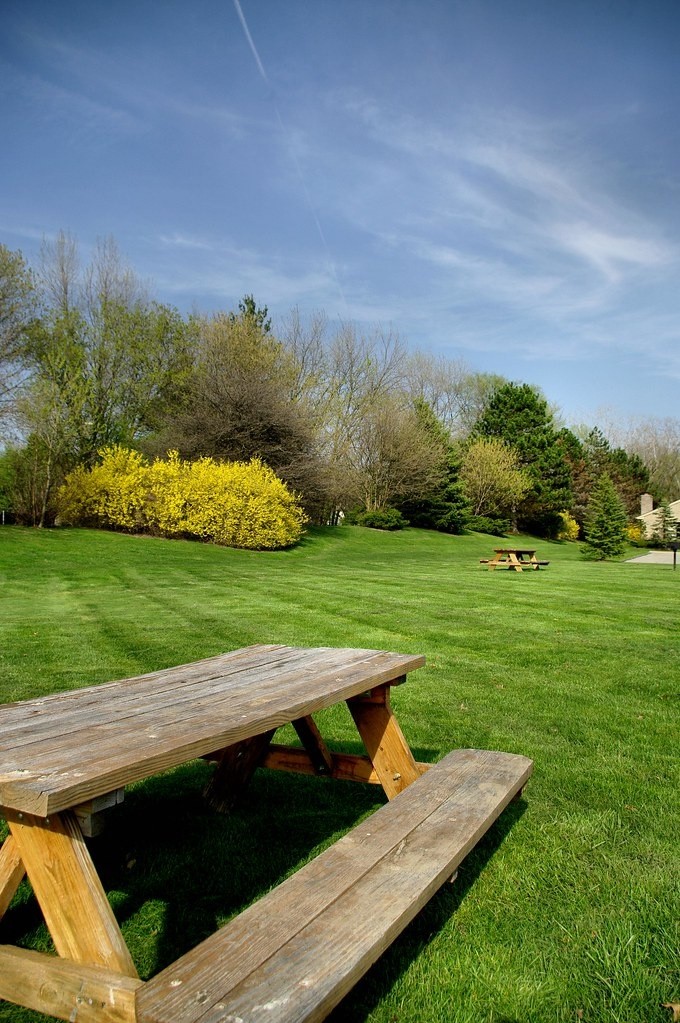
[0,643,420,1022]
[489,548,539,572]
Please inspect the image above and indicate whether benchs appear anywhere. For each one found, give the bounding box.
[479,559,550,571]
[140,748,533,1021]
